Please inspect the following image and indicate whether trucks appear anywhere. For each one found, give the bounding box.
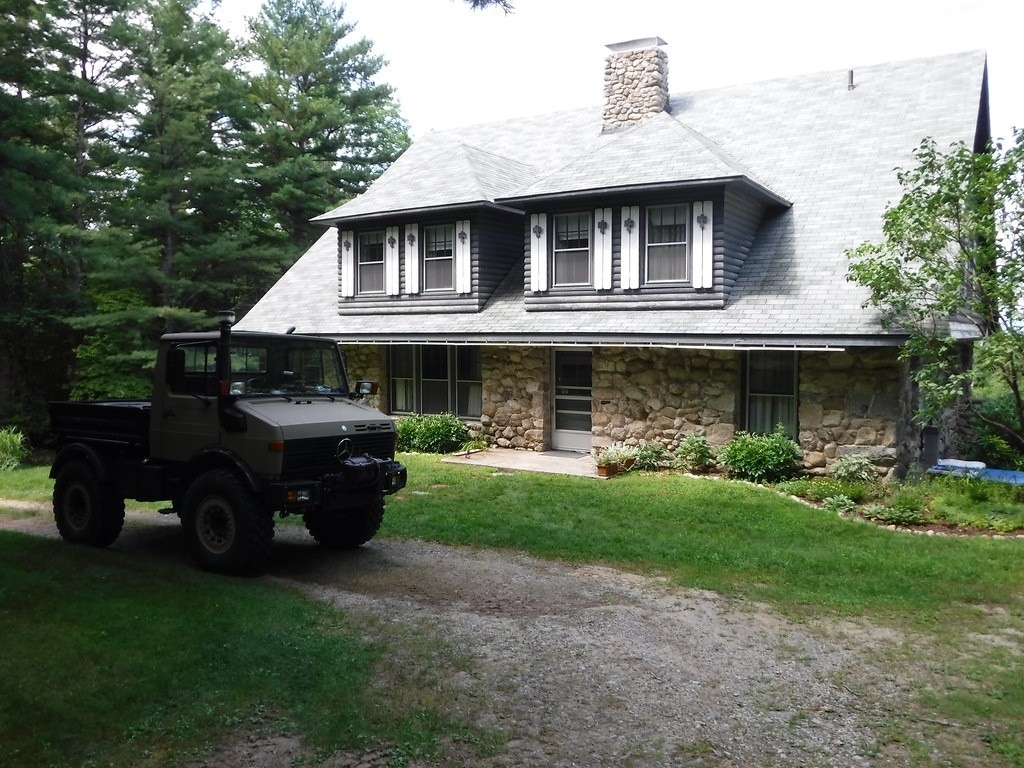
[34,309,409,579]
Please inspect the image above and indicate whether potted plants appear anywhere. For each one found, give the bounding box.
[591,441,639,477]
